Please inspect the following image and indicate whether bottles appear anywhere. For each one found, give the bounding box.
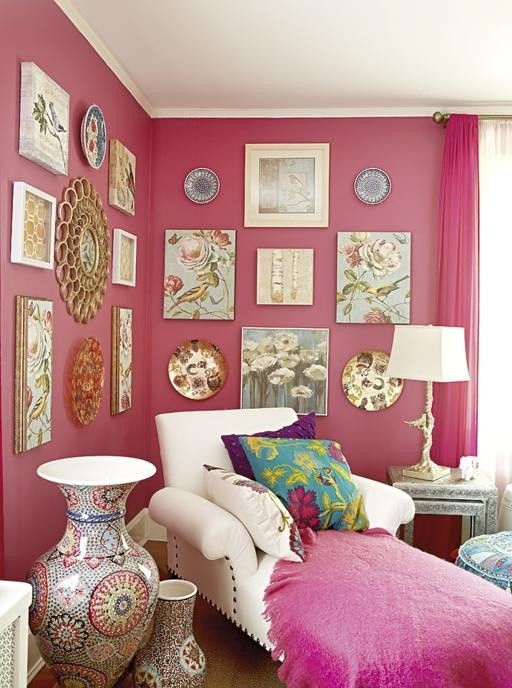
[139,580,207,688]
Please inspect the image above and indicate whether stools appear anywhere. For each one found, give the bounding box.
[454,526,512,590]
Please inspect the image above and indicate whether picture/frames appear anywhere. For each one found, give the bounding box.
[9,178,59,272]
[111,228,139,290]
[240,138,331,230]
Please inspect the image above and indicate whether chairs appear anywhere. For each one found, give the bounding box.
[138,398,510,688]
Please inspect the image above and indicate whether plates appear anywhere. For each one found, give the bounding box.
[185,168,221,204]
[342,351,404,411]
[354,167,392,204]
[168,341,227,400]
[72,336,107,425]
[81,105,108,170]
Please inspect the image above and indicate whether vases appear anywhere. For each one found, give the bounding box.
[133,572,210,688]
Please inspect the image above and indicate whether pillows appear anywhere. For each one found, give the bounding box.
[237,430,367,533]
[218,413,319,481]
[198,453,306,571]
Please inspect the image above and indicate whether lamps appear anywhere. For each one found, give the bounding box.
[383,320,476,481]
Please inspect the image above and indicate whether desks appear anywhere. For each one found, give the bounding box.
[385,465,501,549]
